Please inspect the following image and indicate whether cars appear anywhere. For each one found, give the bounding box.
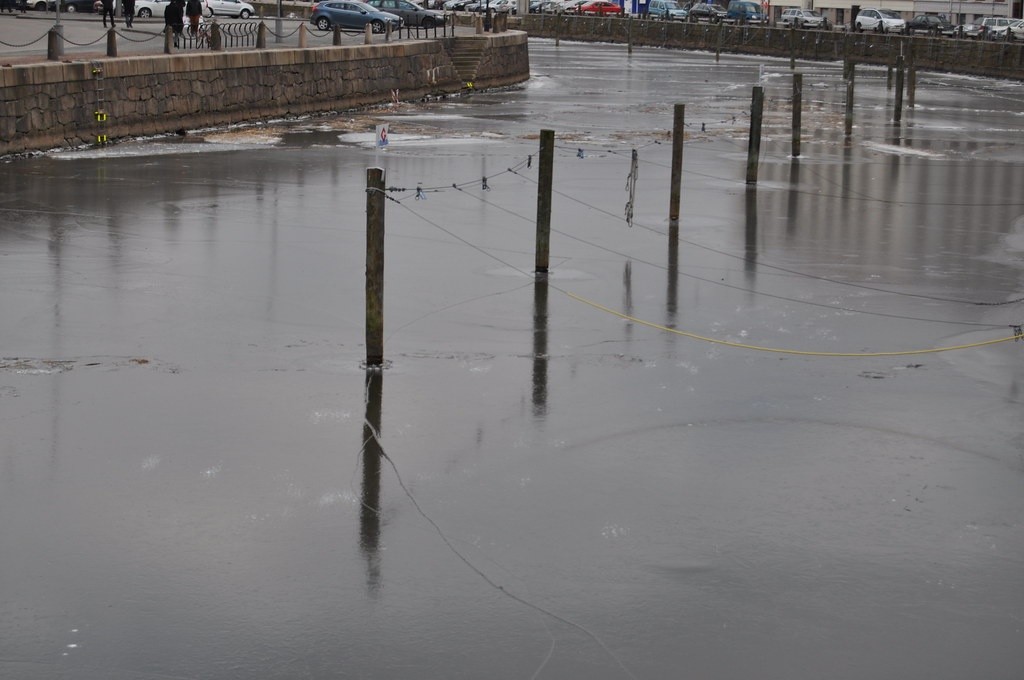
[25,0,63,12]
[781,8,834,29]
[905,13,956,36]
[110,0,189,18]
[58,0,110,13]
[309,0,404,33]
[856,7,907,32]
[415,0,622,16]
[200,0,257,20]
[370,1,450,31]
[956,15,1024,41]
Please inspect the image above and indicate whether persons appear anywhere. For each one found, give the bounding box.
[122,0,136,29]
[101,0,116,28]
[162,0,202,49]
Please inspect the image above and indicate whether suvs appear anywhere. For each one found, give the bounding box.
[647,0,688,21]
[688,2,729,24]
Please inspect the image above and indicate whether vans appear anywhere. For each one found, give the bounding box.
[727,1,770,24]
